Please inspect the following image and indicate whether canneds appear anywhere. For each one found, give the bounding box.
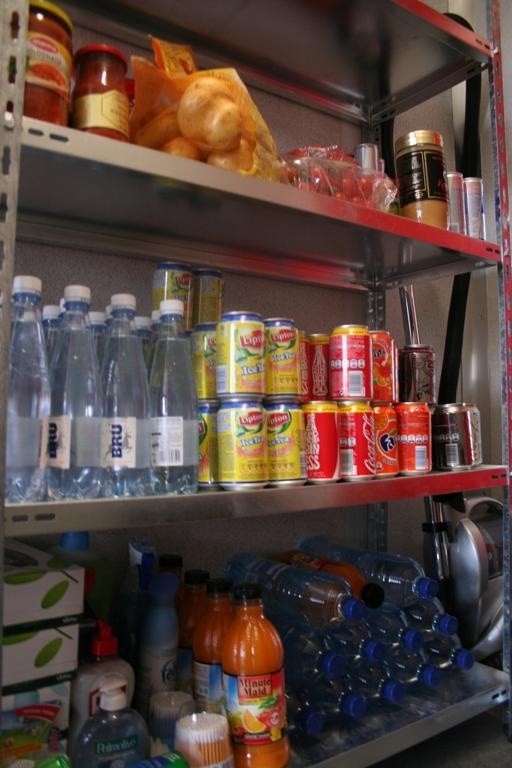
[23,0,74,128]
[71,42,130,142]
[353,129,485,242]
[185,309,483,492]
[193,266,224,328]
[151,260,194,330]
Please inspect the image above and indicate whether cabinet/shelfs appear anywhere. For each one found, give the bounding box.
[0,0,511,768]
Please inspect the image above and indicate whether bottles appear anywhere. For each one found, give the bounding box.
[121,550,290,768]
[226,538,471,736]
[60,528,234,768]
[5,273,200,501]
